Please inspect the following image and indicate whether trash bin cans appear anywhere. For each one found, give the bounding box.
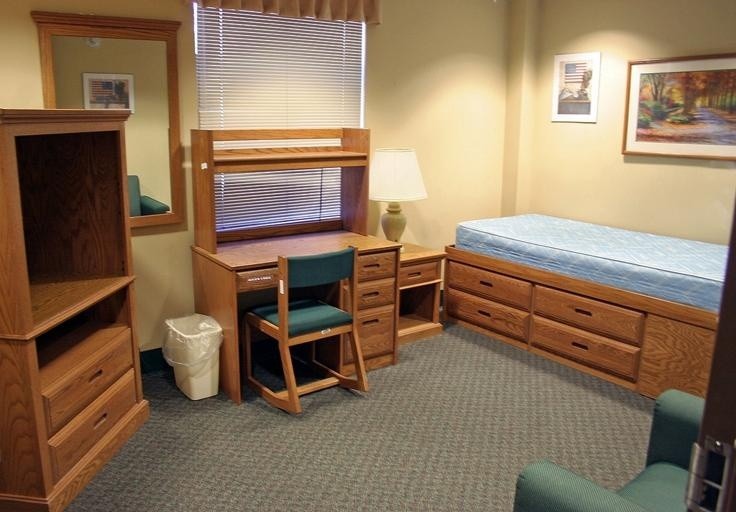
[165,314,222,400]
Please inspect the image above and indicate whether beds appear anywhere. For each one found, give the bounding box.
[444,214,729,402]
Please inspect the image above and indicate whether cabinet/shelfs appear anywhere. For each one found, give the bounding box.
[398,242,448,344]
[191,127,371,255]
[0,108,149,511]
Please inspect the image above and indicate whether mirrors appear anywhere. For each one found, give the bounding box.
[31,10,184,228]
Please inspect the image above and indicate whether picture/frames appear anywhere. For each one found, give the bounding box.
[622,52,736,161]
[82,73,135,111]
[551,52,601,123]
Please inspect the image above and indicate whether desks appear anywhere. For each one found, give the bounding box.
[190,230,403,406]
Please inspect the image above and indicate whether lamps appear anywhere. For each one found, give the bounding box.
[369,149,429,252]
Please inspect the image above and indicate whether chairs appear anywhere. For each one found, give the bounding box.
[127,175,170,217]
[514,388,705,512]
[241,245,370,414]
